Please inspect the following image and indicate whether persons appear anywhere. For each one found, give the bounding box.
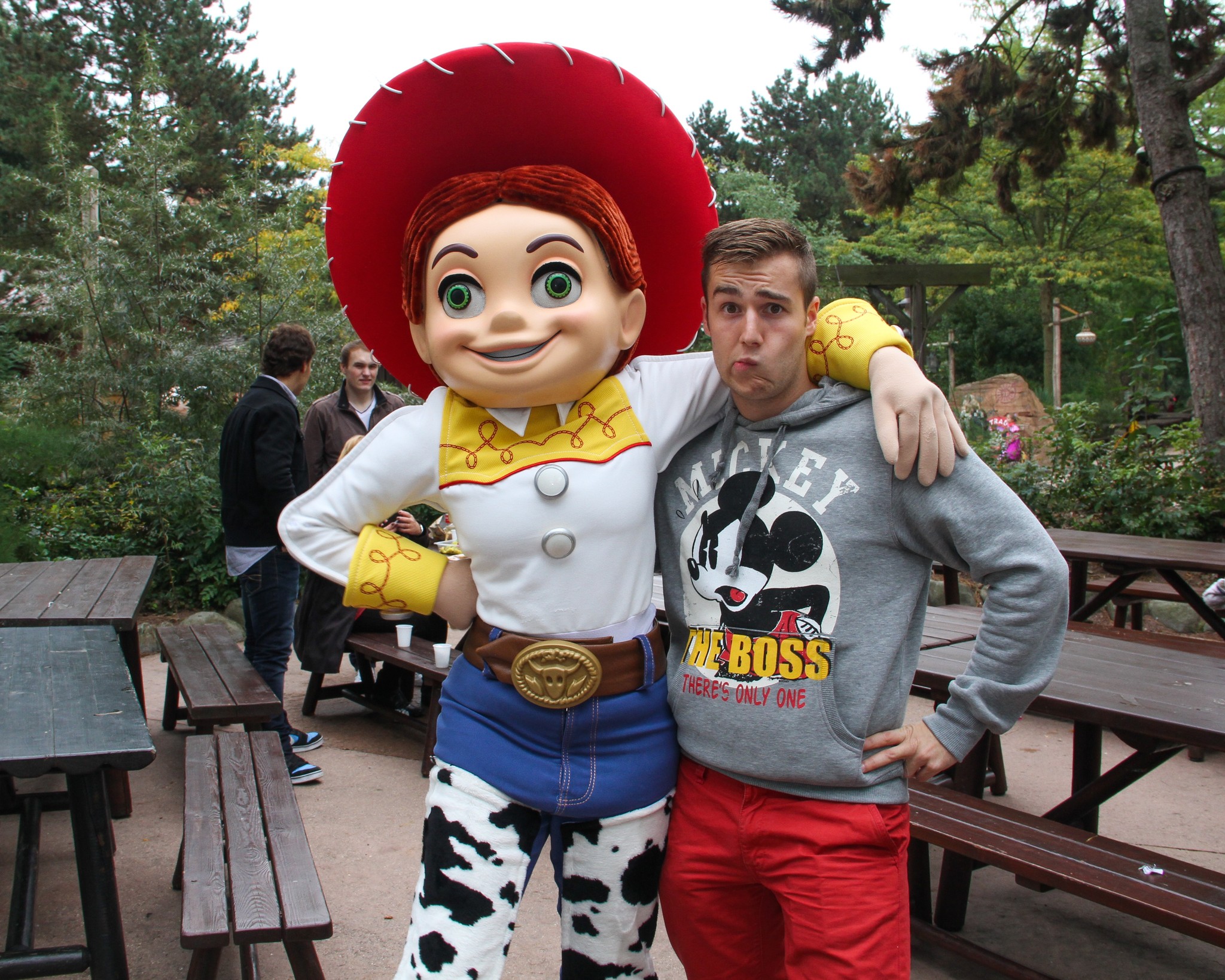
[301,340,406,489]
[654,218,1071,980]
[960,393,1023,466]
[220,324,322,784]
[294,434,448,724]
[276,163,969,980]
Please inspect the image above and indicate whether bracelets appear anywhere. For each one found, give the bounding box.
[419,523,424,535]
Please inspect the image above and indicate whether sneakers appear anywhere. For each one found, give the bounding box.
[289,732,324,754]
[290,757,323,784]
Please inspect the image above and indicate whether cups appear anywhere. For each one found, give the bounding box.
[432,644,452,668]
[396,625,414,647]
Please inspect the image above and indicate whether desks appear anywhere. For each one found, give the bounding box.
[1038,524,1224,630]
[902,629,1224,934]
[1,555,159,729]
[0,626,160,977]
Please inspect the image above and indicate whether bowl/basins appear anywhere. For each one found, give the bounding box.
[434,539,463,554]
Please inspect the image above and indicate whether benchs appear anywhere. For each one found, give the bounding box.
[902,777,1225,980]
[170,728,335,980]
[299,629,463,777]
[940,600,1224,662]
[151,624,284,739]
[1087,575,1207,629]
